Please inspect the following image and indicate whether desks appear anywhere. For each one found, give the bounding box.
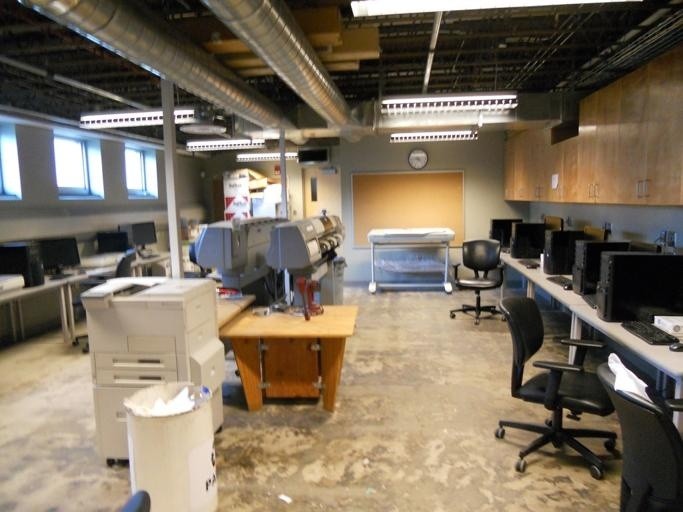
[45,261,138,341]
[500,250,545,298]
[527,271,593,364]
[137,253,170,277]
[368,228,455,294]
[219,303,360,412]
[0,280,72,342]
[569,304,683,433]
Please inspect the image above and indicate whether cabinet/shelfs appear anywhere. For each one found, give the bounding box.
[503,128,576,202]
[577,43,683,205]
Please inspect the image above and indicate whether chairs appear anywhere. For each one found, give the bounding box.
[597,361,683,512]
[495,295,623,479]
[449,239,507,325]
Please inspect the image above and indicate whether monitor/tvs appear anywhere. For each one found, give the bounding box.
[132,222,157,251]
[39,236,80,280]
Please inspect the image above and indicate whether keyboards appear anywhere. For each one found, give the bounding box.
[547,276,572,286]
[621,321,679,344]
[582,294,597,309]
[518,260,540,266]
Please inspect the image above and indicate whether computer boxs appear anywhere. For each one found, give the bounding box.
[0,240,45,289]
[490,219,523,249]
[572,240,629,295]
[544,230,584,274]
[97,231,128,253]
[509,222,550,258]
[596,251,683,322]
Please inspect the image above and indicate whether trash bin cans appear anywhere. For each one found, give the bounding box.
[123,382,218,512]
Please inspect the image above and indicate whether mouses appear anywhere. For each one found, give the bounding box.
[669,343,682,351]
[527,265,536,268]
[564,284,571,290]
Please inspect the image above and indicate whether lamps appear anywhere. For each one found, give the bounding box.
[236,151,299,161]
[351,0,644,18]
[80,105,200,130]
[186,138,266,151]
[381,91,518,117]
[389,124,479,143]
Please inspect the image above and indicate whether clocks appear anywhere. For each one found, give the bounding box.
[409,148,428,169]
